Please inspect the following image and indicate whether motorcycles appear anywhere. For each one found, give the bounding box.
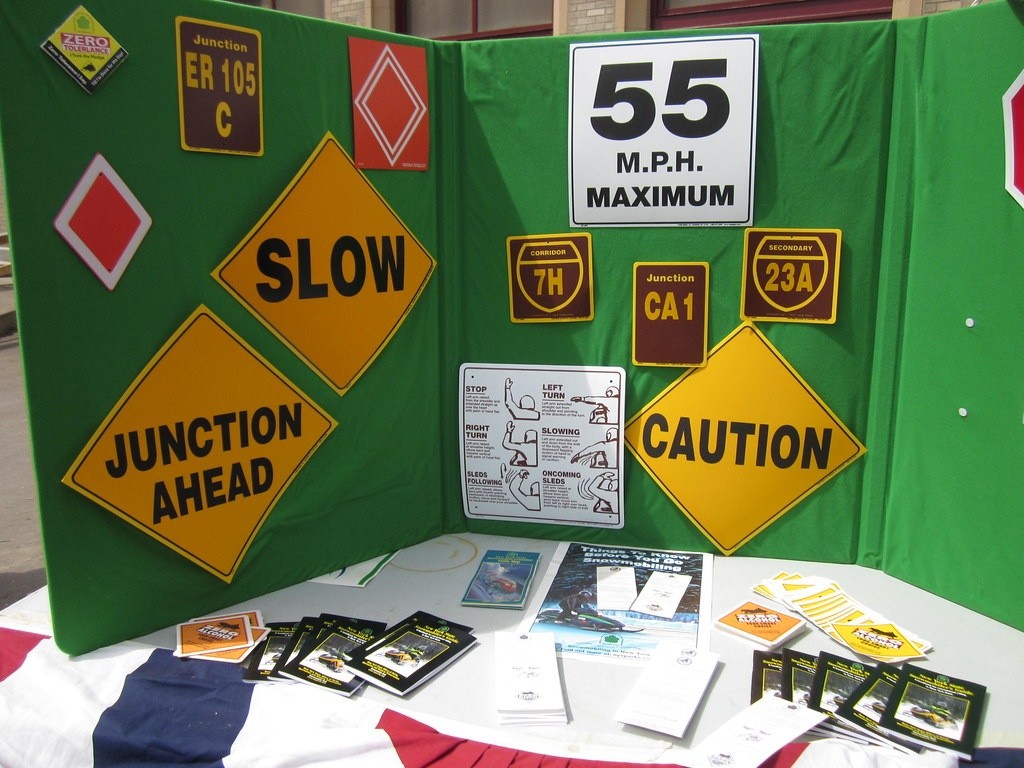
[538,609,645,633]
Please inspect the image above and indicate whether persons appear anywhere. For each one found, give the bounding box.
[555,590,593,623]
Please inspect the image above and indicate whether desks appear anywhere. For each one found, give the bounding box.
[0,535,1024,768]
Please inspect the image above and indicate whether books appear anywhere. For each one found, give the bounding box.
[240,613,477,698]
[749,648,987,762]
